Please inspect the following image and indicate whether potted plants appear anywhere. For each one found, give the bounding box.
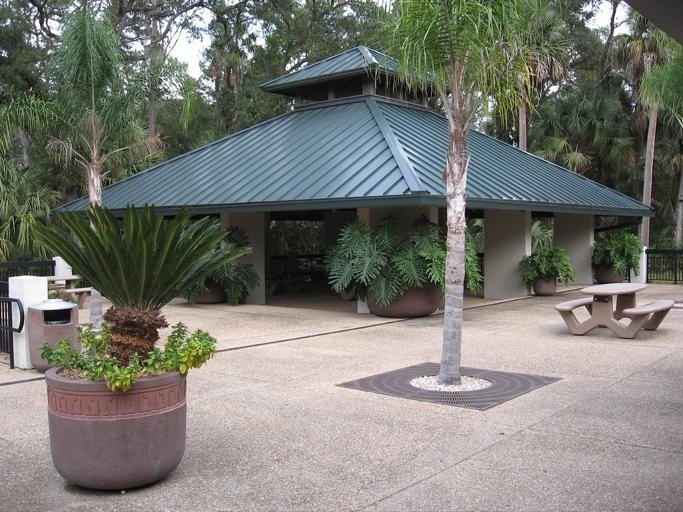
[518,245,577,296]
[591,231,644,282]
[181,224,261,307]
[321,213,483,317]
[28,201,253,494]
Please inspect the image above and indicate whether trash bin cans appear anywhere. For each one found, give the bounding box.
[28,299,82,373]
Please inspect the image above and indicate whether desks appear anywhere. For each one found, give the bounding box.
[580,282,649,339]
[40,275,81,309]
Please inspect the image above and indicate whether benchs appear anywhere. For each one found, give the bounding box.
[622,298,674,339]
[555,297,594,335]
[48,287,91,309]
[48,283,66,289]
